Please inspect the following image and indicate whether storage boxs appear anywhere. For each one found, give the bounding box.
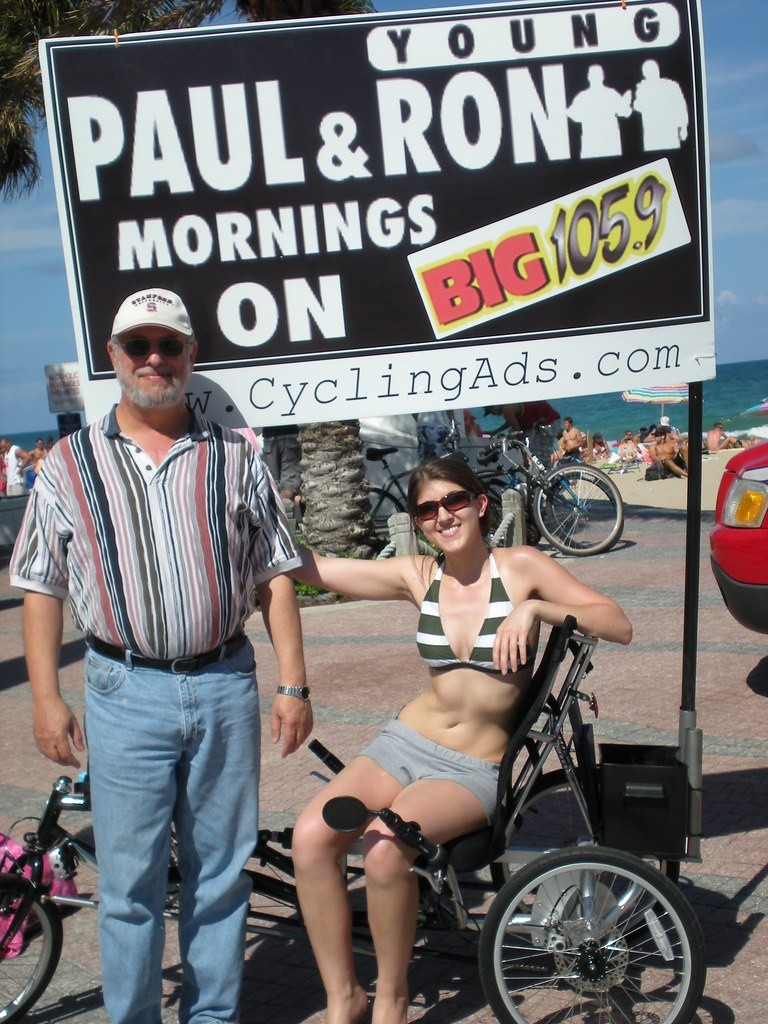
[598,742,690,859]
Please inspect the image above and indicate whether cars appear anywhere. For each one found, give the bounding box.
[709,440,768,637]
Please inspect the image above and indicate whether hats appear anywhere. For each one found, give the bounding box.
[483,405,496,417]
[111,289,196,341]
[650,430,666,436]
[660,416,670,427]
[463,411,477,420]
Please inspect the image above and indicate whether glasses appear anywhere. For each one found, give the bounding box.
[414,491,471,520]
[718,426,722,430]
[120,337,185,356]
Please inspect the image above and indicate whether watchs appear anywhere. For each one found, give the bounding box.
[277,685,311,701]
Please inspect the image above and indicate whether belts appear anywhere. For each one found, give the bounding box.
[87,631,247,674]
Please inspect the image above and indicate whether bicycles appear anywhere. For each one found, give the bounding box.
[365,420,625,559]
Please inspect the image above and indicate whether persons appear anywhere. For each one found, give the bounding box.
[292,460,632,1024]
[463,401,561,471]
[551,417,761,481]
[9,288,314,1024]
[1,432,54,496]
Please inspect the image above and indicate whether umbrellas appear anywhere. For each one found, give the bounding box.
[622,382,688,418]
[741,398,768,416]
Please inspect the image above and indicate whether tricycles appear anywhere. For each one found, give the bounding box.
[0,606,708,1024]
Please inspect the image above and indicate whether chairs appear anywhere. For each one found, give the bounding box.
[437,616,599,877]
[639,442,654,464]
[599,450,639,476]
[583,430,593,468]
